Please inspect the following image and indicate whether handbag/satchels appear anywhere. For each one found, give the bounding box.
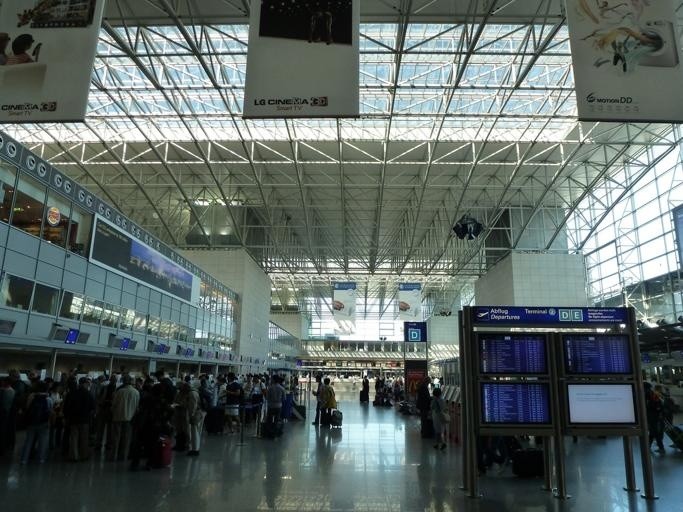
[439,411,451,424]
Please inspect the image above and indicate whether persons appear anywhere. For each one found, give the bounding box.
[642,381,673,452]
[0,368,301,472]
[310,376,336,427]
[416,375,450,450]
[360,374,406,408]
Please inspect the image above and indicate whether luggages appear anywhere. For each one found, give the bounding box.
[204,398,225,436]
[330,402,342,429]
[660,415,683,451]
[159,436,171,465]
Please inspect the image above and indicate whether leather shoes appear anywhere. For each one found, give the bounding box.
[440,444,446,449]
[433,444,438,448]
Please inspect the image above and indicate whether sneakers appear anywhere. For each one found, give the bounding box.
[186,450,199,456]
